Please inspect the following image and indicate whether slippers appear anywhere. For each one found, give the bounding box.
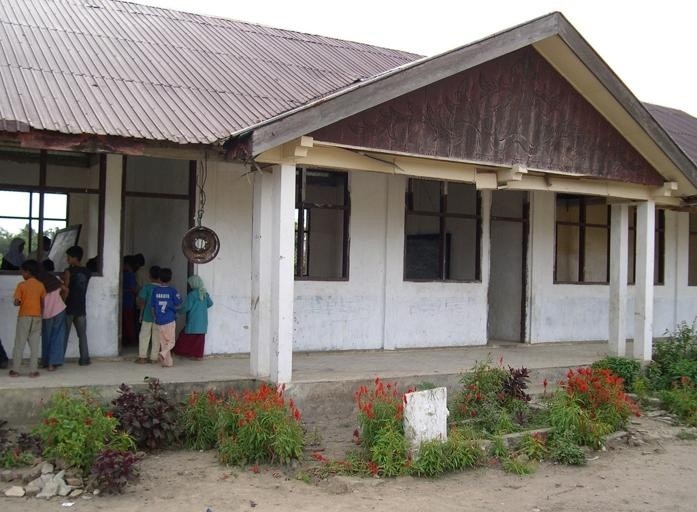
[8,364,57,378]
[133,358,156,364]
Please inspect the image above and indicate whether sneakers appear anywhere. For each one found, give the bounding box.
[79,359,91,366]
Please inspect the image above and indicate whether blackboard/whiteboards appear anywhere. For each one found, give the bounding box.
[48,223,82,273]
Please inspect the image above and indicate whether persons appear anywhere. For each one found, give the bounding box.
[39,259,70,371]
[60,245,92,366]
[26,236,51,264]
[121,255,138,347]
[132,265,161,364]
[171,274,213,361]
[7,259,48,379]
[1,237,26,270]
[150,268,183,368]
[127,253,150,291]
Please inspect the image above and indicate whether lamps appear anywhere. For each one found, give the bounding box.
[181,150,220,264]
